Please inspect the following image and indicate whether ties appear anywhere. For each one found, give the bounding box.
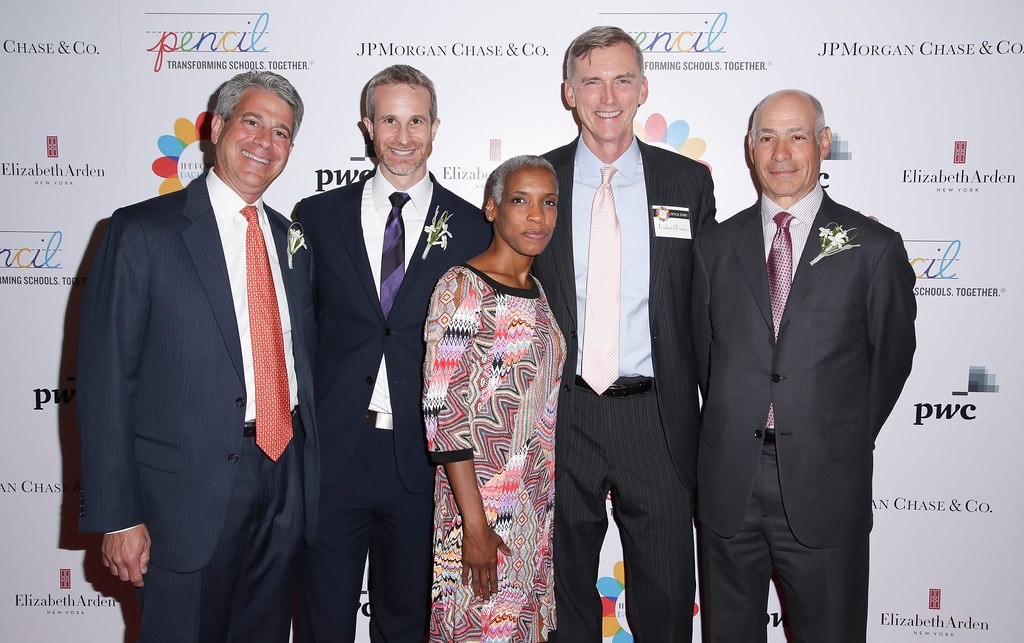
[380,193,411,323]
[240,206,294,463]
[582,165,620,396]
[766,212,798,429]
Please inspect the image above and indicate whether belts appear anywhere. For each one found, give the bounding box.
[363,410,394,431]
[244,407,299,438]
[762,429,777,445]
[575,375,652,398]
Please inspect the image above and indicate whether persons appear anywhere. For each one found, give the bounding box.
[72,25,918,643]
[422,155,568,643]
